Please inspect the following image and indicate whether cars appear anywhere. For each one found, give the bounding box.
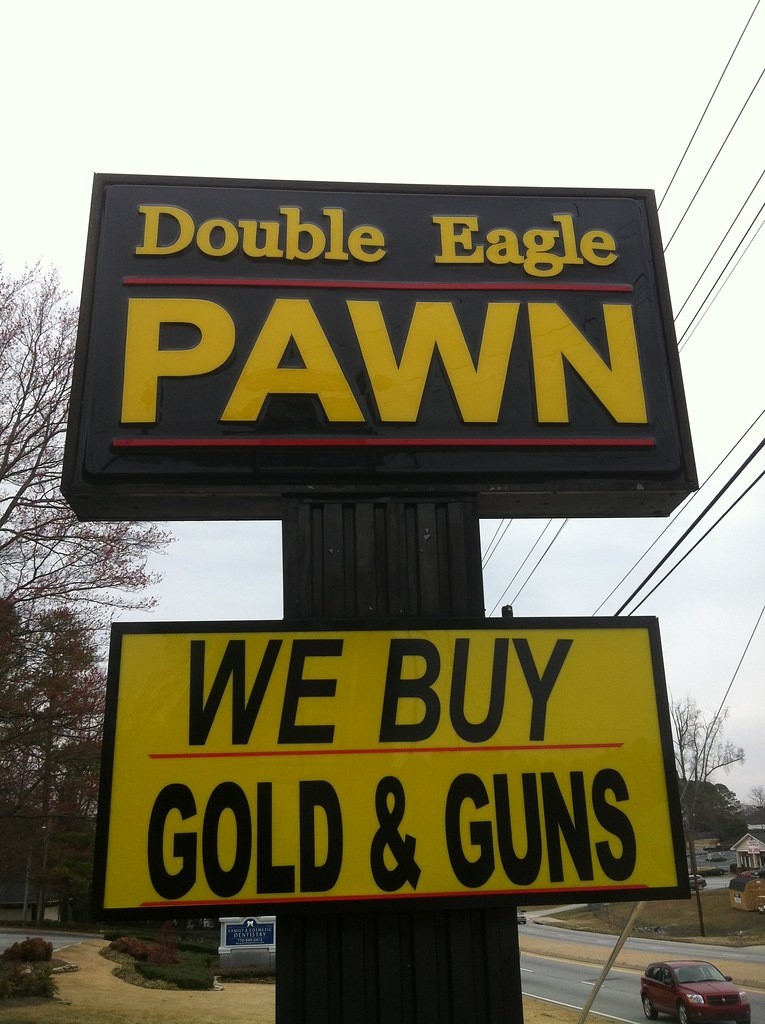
[736,865,765,879]
[640,960,751,1024]
[689,875,707,891]
[516,912,526,924]
